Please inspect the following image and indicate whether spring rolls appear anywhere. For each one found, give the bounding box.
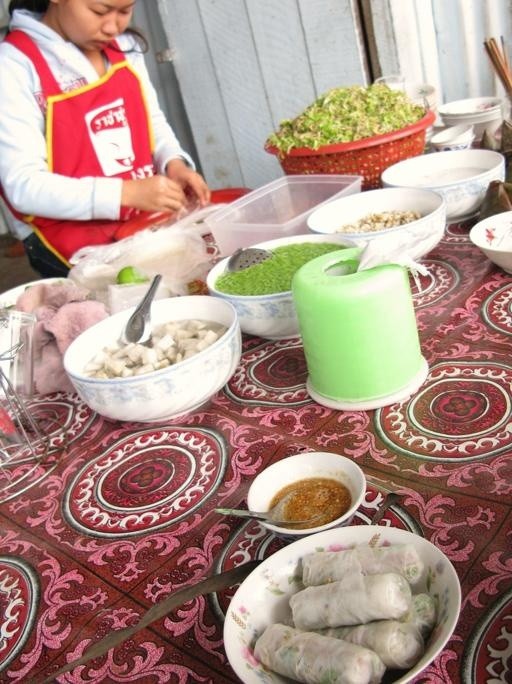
[252,545,441,683]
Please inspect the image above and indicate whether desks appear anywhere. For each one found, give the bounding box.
[0,131,512,679]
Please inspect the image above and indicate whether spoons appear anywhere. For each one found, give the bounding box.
[120,276,168,349]
[208,489,297,527]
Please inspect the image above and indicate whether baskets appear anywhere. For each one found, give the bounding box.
[263,104,436,198]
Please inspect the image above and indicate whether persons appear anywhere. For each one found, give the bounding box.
[0,0,253,276]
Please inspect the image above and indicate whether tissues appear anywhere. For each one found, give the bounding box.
[291,222,444,409]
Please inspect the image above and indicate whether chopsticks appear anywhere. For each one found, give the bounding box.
[481,35,512,105]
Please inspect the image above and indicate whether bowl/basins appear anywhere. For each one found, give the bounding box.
[205,231,362,343]
[377,150,507,225]
[111,186,256,256]
[0,276,77,330]
[466,209,512,276]
[422,96,505,150]
[223,522,464,684]
[245,449,369,542]
[304,186,444,272]
[59,296,245,429]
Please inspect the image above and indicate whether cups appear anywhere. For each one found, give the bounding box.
[371,76,407,93]
[0,307,41,405]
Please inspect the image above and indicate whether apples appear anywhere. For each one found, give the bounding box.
[116,266,151,285]
